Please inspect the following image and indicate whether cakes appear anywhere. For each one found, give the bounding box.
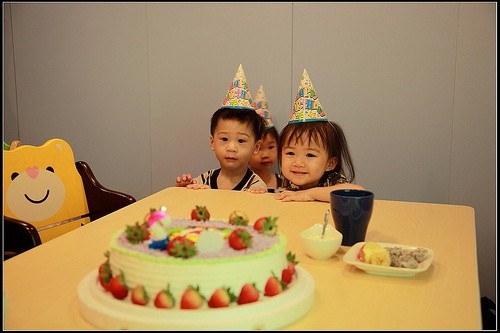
[89,207,303,314]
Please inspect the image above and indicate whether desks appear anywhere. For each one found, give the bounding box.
[2,187,483,331]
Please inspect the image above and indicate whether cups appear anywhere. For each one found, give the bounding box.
[330,189,374,246]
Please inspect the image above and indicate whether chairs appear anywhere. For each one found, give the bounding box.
[2,137,136,262]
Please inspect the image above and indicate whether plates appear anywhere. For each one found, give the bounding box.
[343,242,434,277]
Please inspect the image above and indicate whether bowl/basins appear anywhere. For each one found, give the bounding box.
[299,223,343,260]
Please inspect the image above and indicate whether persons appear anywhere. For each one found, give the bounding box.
[246,86,284,193]
[274,69,365,203]
[177,63,268,193]
